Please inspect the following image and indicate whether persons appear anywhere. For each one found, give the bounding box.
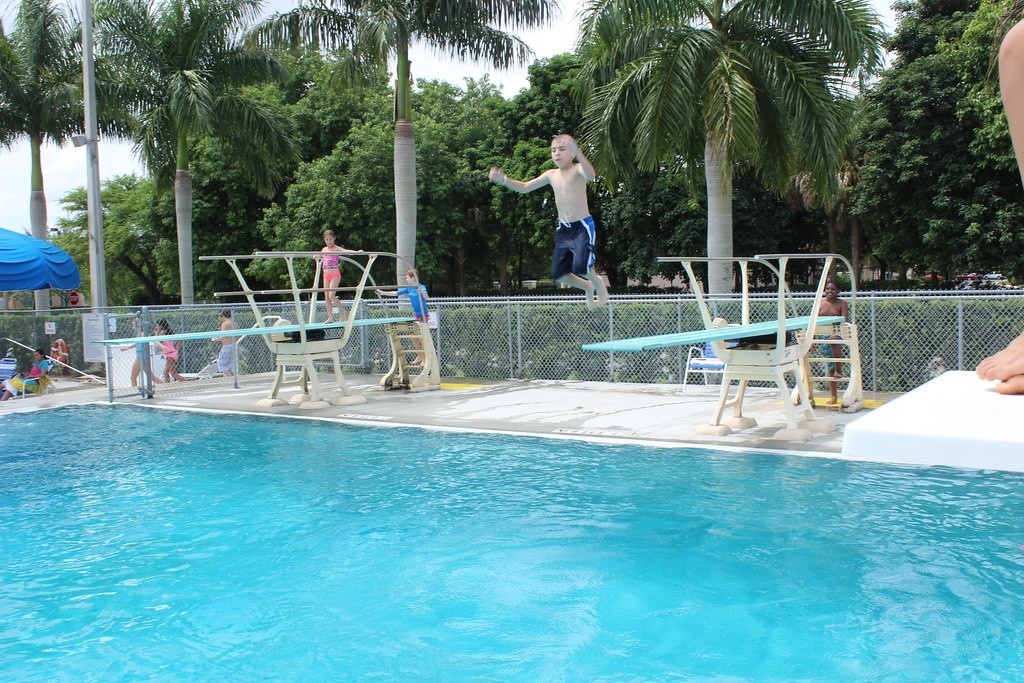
[314,230,364,324]
[0,349,48,401]
[490,134,607,310]
[211,310,240,376]
[976,19,1024,395]
[374,270,428,367]
[120,316,185,388]
[810,283,849,404]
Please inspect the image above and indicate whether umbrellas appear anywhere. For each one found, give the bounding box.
[0,227,81,291]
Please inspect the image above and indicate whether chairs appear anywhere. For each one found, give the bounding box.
[0,357,55,400]
[682,324,740,393]
[177,359,225,381]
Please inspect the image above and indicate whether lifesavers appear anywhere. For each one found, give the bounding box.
[51,339,69,366]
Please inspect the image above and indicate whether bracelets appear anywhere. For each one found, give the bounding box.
[502,174,507,185]
